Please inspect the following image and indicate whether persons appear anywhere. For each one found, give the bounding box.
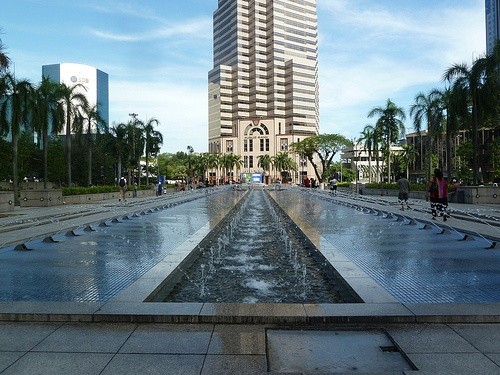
[119,174,127,202]
[397,173,411,211]
[305,177,319,189]
[440,173,450,218]
[175,177,214,192]
[428,169,447,222]
[154,182,167,196]
[134,181,138,195]
[330,177,338,193]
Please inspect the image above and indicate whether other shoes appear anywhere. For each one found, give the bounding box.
[119,199,121,201]
[443,215,447,222]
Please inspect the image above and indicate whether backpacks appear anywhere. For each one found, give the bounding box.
[119,179,125,187]
